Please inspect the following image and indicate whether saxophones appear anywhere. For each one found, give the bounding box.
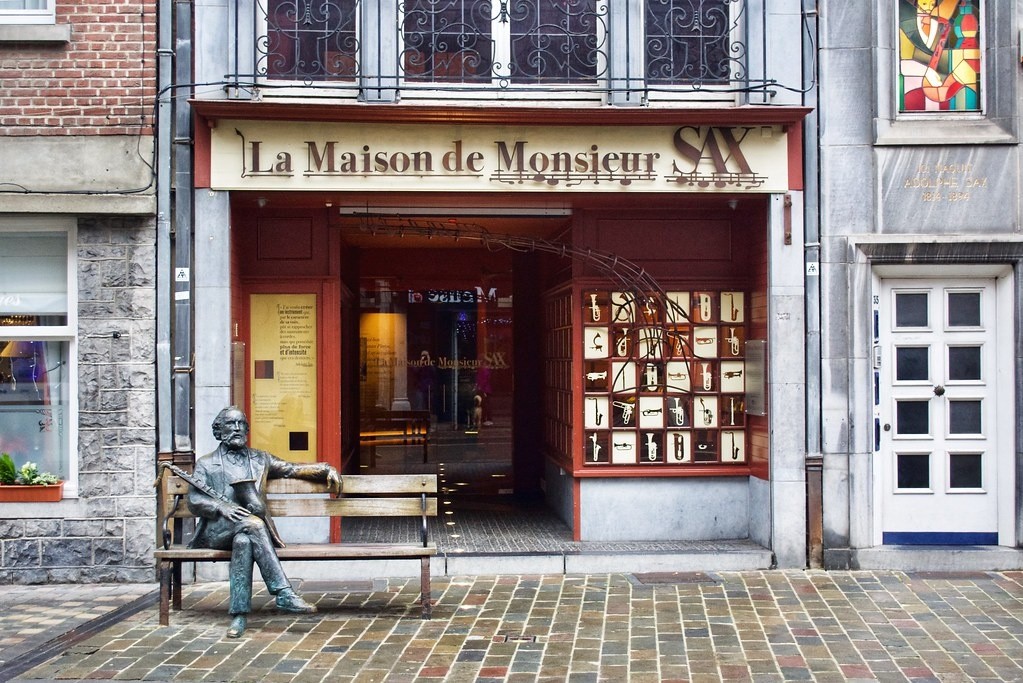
[151,459,240,508]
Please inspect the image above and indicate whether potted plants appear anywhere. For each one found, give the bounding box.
[0,452,64,502]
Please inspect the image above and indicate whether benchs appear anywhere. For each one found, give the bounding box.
[154,475,438,625]
[360,410,430,467]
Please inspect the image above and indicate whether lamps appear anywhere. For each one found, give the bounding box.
[0,341,35,358]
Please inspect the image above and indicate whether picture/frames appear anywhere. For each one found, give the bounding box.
[890,0,987,120]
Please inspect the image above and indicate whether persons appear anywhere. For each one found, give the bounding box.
[186,406,343,638]
[475,354,494,427]
[413,351,446,434]
